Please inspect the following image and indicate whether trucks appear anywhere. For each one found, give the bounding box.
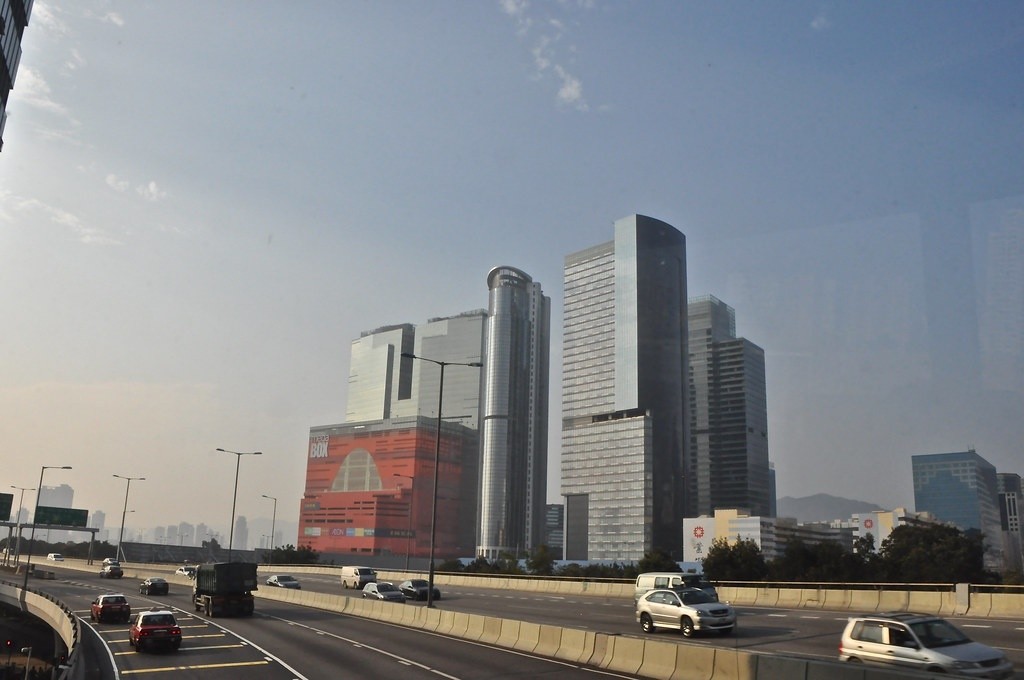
[340,566,377,590]
[3,548,15,555]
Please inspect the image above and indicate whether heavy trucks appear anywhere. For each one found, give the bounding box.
[187,562,259,618]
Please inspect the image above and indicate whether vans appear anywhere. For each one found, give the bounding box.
[633,572,719,606]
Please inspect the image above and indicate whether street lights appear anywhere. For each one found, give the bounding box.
[400,352,484,609]
[215,447,263,566]
[21,646,33,680]
[261,535,269,549]
[206,533,218,539]
[176,534,189,546]
[24,465,73,589]
[262,495,277,564]
[393,472,416,570]
[159,536,172,545]
[10,485,36,566]
[112,474,145,562]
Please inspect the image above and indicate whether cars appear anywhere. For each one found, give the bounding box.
[128,606,183,652]
[635,588,737,639]
[175,565,198,575]
[140,577,169,596]
[361,582,407,604]
[47,552,64,562]
[99,565,124,578]
[398,579,440,601]
[90,591,130,624]
[102,558,119,567]
[837,611,1015,680]
[266,574,302,589]
